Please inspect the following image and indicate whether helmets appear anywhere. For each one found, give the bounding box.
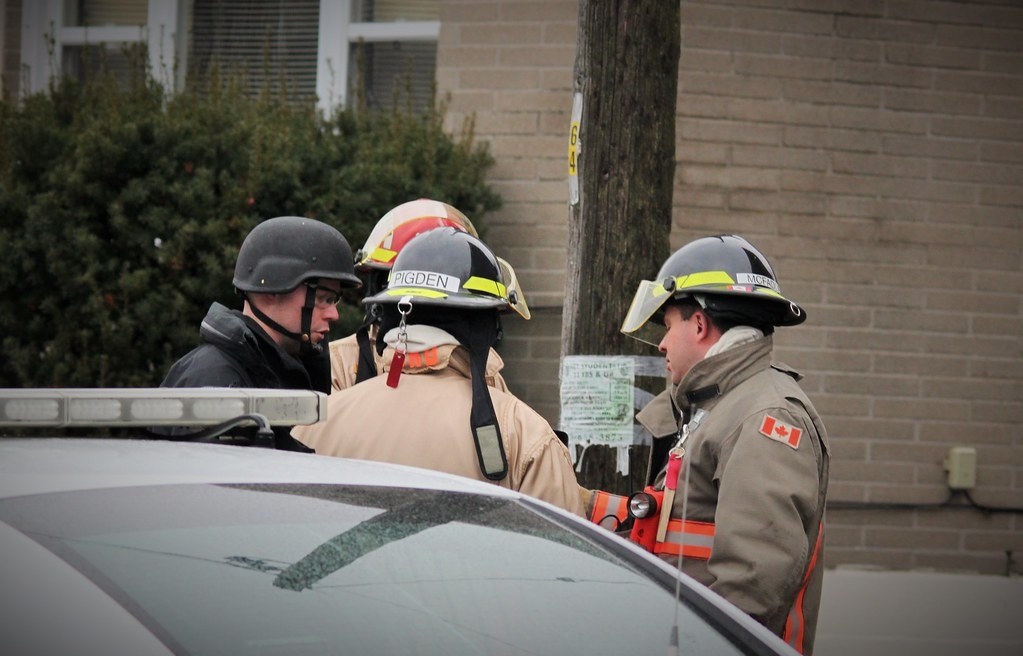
[233,216,363,292]
[648,235,807,347]
[362,226,512,308]
[354,198,480,272]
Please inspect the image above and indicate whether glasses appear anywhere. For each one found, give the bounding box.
[304,282,342,310]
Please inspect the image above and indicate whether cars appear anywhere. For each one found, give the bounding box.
[0,387,815,656]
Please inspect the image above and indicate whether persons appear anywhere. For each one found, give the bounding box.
[289,226,587,521]
[581,234,832,656]
[328,199,480,393]
[146,216,363,454]
